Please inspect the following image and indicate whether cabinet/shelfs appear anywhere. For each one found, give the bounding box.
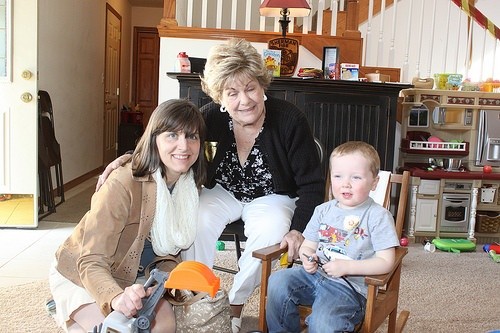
[167,73,414,178]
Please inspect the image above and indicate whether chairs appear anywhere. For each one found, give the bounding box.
[38,91,65,215]
[253,171,410,333]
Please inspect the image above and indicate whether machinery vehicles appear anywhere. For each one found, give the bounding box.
[99,259,222,333]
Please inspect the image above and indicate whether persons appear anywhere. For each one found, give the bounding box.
[49,98,209,333]
[266,141,400,333]
[94,37,325,333]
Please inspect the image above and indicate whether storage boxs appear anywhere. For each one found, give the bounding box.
[340,63,359,81]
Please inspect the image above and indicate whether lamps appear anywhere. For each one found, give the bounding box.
[259,0,311,79]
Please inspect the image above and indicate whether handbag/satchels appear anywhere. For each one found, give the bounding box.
[145,256,233,333]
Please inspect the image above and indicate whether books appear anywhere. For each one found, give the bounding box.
[262,48,281,78]
[340,63,359,81]
[299,68,323,78]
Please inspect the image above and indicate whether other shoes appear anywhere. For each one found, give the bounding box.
[232,316,242,333]
